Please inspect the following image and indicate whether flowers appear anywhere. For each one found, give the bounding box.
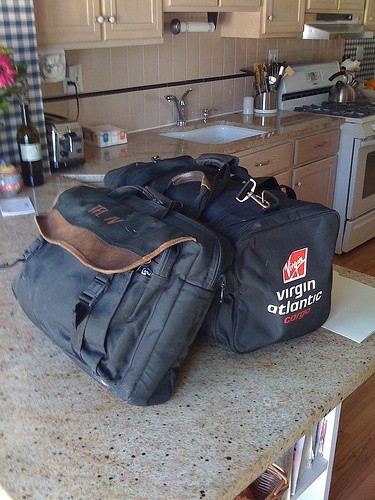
[0,42,28,116]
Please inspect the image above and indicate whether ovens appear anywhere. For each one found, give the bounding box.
[332,122,375,255]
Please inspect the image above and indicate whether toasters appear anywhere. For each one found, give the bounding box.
[44,112,85,170]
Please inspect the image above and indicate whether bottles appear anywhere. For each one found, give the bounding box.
[242,96,254,114]
[17,99,44,188]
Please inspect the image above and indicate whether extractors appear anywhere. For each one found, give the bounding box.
[303,12,374,40]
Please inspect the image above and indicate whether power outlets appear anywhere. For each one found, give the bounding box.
[63,64,82,94]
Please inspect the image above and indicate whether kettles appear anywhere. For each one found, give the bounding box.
[328,71,360,103]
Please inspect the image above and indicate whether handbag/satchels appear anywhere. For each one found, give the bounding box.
[102,153,340,354]
[11,186,234,406]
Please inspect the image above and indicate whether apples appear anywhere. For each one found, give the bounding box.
[364,78,375,91]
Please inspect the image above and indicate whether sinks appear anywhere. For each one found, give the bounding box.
[157,123,270,145]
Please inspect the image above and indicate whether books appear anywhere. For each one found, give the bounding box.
[0,197,35,217]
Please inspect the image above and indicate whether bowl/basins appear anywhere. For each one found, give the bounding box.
[356,86,375,99]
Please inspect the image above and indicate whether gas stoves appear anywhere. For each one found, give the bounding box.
[276,60,375,124]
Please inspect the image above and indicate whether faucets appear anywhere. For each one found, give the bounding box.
[166,87,192,127]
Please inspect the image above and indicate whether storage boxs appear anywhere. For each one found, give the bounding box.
[82,122,128,148]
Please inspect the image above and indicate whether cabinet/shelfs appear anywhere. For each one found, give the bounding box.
[275,402,342,500]
[33,0,375,51]
[230,127,340,209]
